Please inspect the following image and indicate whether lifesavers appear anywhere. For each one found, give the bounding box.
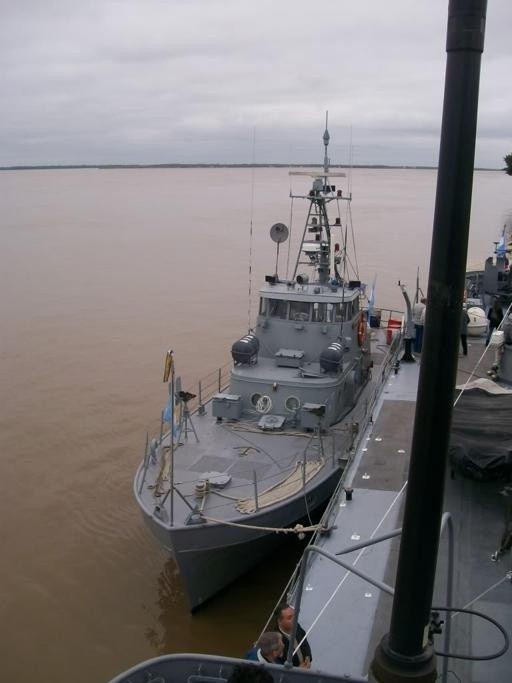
[358,317,367,345]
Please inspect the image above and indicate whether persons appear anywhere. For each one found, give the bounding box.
[241,629,311,672]
[459,306,470,357]
[485,312,512,383]
[413,296,427,353]
[485,296,503,347]
[266,602,312,672]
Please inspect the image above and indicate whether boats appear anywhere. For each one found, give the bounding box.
[126,103,404,618]
[104,0,512,683]
